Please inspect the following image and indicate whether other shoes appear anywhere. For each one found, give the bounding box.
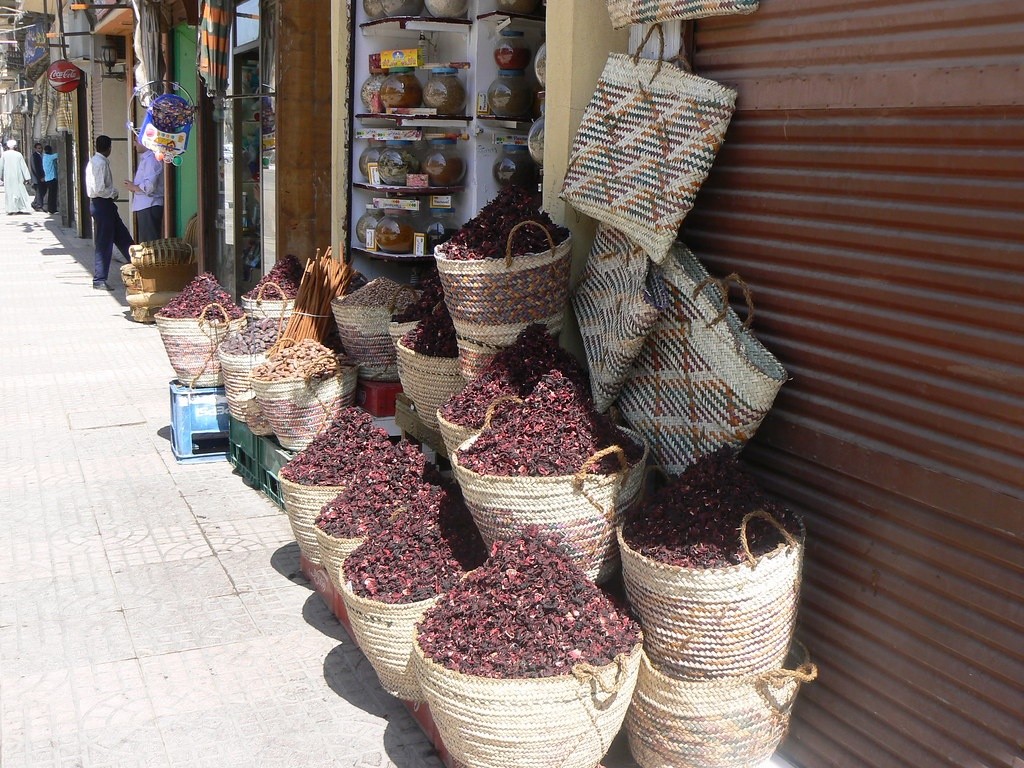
[92,280,114,291]
[48,210,59,215]
[35,208,46,212]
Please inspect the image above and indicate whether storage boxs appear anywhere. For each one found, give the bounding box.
[168,377,437,515]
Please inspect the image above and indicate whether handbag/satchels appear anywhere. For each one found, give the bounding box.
[568,221,667,412]
[619,243,788,474]
[557,22,737,265]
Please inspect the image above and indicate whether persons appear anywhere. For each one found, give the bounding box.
[253,183,260,203]
[0,139,31,215]
[123,133,164,244]
[85,135,136,291]
[31,143,60,214]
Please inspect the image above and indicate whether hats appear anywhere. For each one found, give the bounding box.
[7,140,17,148]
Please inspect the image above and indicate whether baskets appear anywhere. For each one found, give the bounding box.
[152,218,821,768]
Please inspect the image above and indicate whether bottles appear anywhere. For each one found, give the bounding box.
[357,1,548,257]
[216,190,250,234]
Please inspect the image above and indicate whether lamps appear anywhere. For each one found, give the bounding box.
[101,45,126,78]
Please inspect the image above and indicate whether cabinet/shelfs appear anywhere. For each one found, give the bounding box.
[476,8,546,130]
[349,13,472,265]
